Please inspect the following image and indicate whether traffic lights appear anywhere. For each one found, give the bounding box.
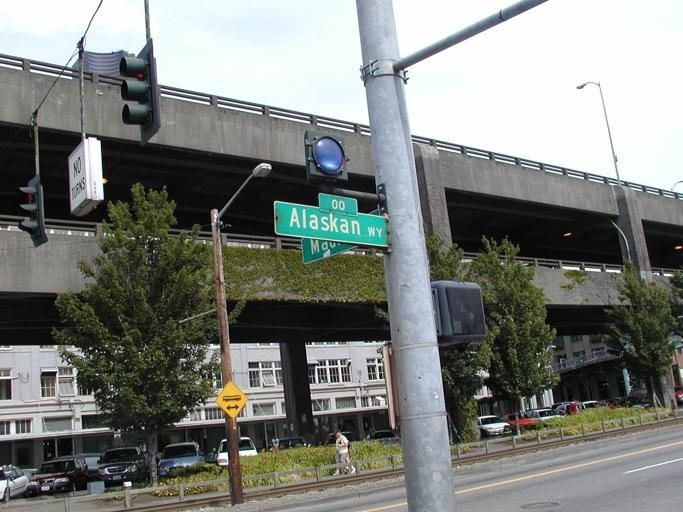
[118,38,162,147]
[18,173,49,248]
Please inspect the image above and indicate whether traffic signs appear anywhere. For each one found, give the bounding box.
[273,191,389,264]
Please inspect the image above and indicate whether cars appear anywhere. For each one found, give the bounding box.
[212,436,258,467]
[278,429,402,451]
[475,398,600,438]
[27,455,90,498]
[158,441,202,477]
[0,464,28,502]
[96,446,146,483]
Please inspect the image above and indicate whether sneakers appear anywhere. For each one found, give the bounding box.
[332,470,339,475]
[349,465,356,473]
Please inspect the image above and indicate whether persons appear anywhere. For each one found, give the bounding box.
[568,398,581,416]
[331,430,356,475]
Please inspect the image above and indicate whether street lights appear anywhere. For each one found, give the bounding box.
[209,161,273,507]
[576,80,620,180]
